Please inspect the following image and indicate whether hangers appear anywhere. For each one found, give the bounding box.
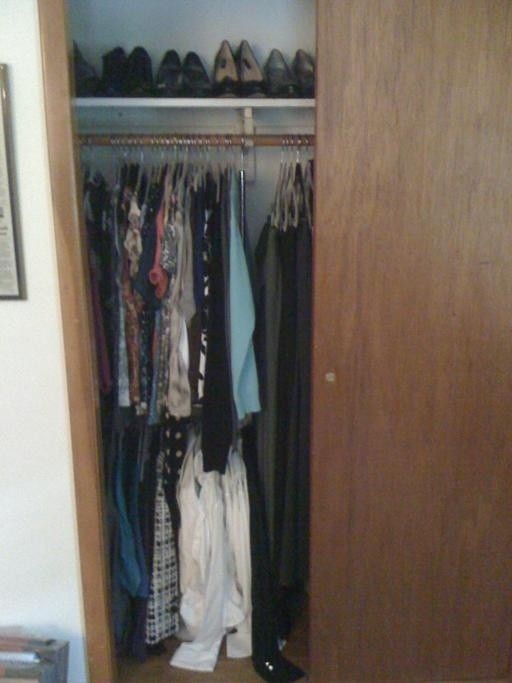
[76,128,317,231]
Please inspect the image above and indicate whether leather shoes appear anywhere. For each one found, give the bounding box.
[125,45,153,97]
[211,38,243,98]
[152,48,184,97]
[263,48,299,98]
[95,46,125,97]
[291,49,315,98]
[181,50,213,98]
[234,39,267,98]
